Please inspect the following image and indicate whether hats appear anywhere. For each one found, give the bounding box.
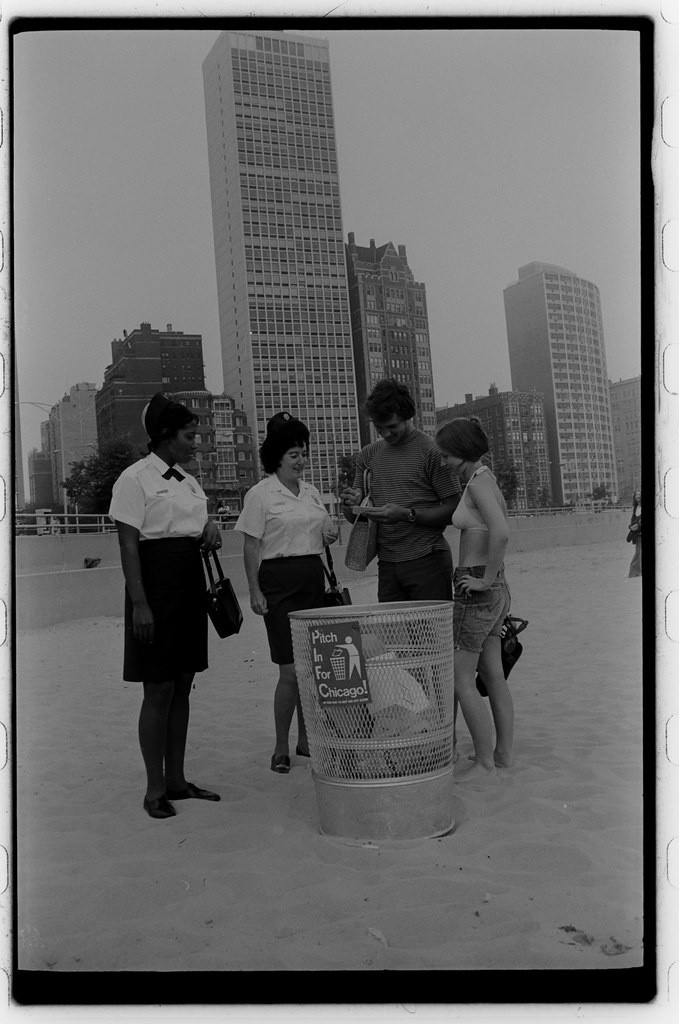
[142,392,173,440]
[267,411,293,437]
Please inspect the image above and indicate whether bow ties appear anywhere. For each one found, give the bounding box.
[162,467,186,482]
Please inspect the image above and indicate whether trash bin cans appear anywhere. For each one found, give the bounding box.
[287,600,456,843]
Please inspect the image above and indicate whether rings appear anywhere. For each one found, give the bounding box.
[216,542,221,545]
[343,499,349,506]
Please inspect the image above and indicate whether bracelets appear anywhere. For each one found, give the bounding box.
[481,580,494,591]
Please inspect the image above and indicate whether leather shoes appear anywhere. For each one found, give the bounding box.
[166,784,220,800]
[144,794,176,818]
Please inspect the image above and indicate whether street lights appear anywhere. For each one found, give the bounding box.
[192,452,216,491]
[231,485,250,513]
[52,447,81,533]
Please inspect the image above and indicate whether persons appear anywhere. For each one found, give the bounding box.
[626,488,642,578]
[218,499,231,530]
[434,417,514,786]
[108,391,223,818]
[340,379,464,755]
[234,411,340,774]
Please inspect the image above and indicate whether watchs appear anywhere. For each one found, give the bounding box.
[408,507,416,523]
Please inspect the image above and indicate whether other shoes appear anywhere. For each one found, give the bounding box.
[296,745,311,758]
[271,752,290,772]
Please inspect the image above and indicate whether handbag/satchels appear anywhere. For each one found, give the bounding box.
[319,545,352,607]
[626,530,635,542]
[201,544,244,639]
[476,609,529,696]
[344,467,378,572]
